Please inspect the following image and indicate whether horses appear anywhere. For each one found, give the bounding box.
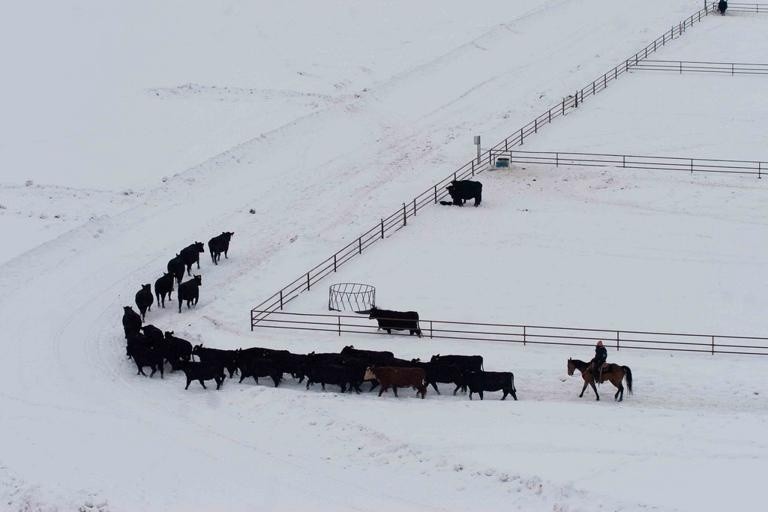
[568,357,632,402]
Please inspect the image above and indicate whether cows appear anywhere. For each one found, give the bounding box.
[450,180,483,204]
[718,0,727,15]
[446,184,480,207]
[125,324,195,379]
[208,231,234,265]
[135,284,153,323]
[170,343,518,400]
[368,305,421,336]
[155,240,206,313]
[122,306,142,336]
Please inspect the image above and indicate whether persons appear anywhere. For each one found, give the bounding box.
[590,341,607,382]
